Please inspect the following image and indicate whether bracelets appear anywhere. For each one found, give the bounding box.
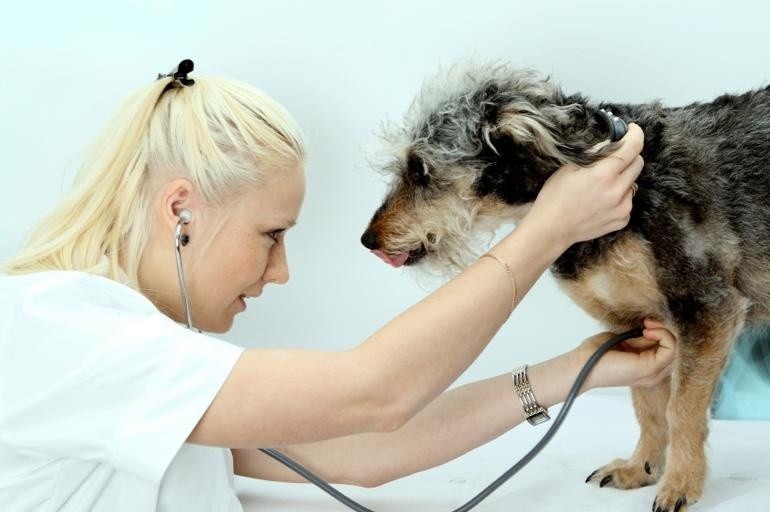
[481,252,516,318]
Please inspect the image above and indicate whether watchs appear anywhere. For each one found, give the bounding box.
[513,365,550,428]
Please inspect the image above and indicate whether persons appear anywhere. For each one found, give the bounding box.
[2,72,677,512]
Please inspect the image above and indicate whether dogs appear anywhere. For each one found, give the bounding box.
[360,56,769,512]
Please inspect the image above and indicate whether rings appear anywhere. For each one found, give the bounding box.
[631,185,639,198]
